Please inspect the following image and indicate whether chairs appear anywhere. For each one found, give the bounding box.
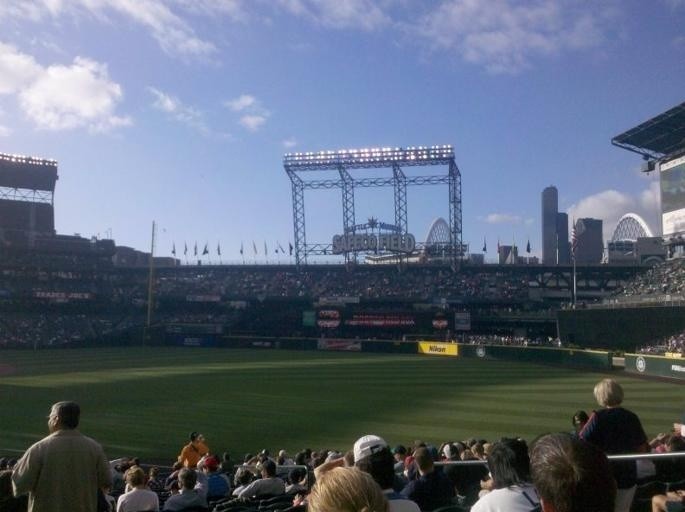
[99,460,685,512]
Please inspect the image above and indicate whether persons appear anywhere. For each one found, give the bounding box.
[0,256,685,354]
[1,379,684,512]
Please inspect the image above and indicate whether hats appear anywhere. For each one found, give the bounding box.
[205,458,217,466]
[573,411,587,424]
[353,435,386,462]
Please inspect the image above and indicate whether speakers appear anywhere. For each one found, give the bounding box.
[639,161,655,172]
